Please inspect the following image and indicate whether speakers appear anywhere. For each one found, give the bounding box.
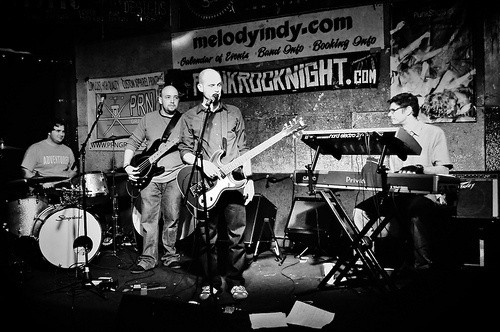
[284,197,342,246]
[430,172,498,220]
[241,193,277,250]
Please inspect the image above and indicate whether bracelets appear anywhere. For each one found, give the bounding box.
[247,176,252,180]
[124,165,129,170]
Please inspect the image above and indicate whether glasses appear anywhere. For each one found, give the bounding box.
[389,107,405,113]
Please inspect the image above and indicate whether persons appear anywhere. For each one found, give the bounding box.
[124,85,188,274]
[21,119,77,179]
[177,69,255,298]
[353,93,453,238]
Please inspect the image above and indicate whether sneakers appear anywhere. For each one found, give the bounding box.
[230,285,248,299]
[200,286,218,299]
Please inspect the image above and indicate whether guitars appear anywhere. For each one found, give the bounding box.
[175,116,308,212]
[125,134,180,190]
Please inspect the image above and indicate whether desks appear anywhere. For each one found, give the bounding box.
[294,183,430,288]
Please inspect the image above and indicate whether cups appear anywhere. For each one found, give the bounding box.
[140,283,147,295]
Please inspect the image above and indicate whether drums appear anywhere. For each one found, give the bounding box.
[4,195,52,240]
[71,171,110,201]
[32,203,104,270]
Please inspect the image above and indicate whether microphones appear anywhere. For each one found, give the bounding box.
[205,94,219,105]
[98,93,106,112]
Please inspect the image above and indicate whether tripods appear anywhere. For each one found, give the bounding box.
[48,111,115,299]
[160,107,223,299]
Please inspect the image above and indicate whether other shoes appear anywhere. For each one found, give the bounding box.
[168,261,182,268]
[129,265,145,273]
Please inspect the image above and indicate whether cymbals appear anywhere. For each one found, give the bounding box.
[9,175,70,185]
[91,135,131,144]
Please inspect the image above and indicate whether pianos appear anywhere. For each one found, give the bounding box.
[294,127,461,298]
